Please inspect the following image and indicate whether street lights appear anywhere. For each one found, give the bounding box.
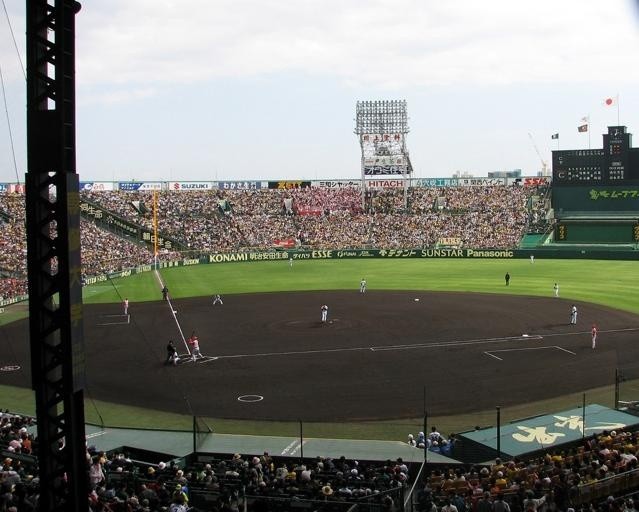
[357,98,409,207]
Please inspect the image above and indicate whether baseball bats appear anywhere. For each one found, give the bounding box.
[190,331,195,339]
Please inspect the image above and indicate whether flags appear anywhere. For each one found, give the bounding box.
[551,133,559,140]
[601,96,618,107]
[580,117,588,123]
[577,124,588,132]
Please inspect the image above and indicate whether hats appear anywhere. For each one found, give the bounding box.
[148,461,183,476]
[408,431,447,445]
[611,430,632,436]
[234,453,241,459]
[252,456,260,463]
[4,457,12,461]
[479,461,515,477]
[322,485,333,495]
[21,427,26,432]
[351,468,358,475]
[176,484,181,490]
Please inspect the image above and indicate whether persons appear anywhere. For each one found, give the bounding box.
[163,341,175,364]
[553,283,558,296]
[212,294,224,305]
[169,346,181,365]
[591,324,597,349]
[570,304,576,325]
[321,303,329,323]
[123,297,129,314]
[188,336,205,361]
